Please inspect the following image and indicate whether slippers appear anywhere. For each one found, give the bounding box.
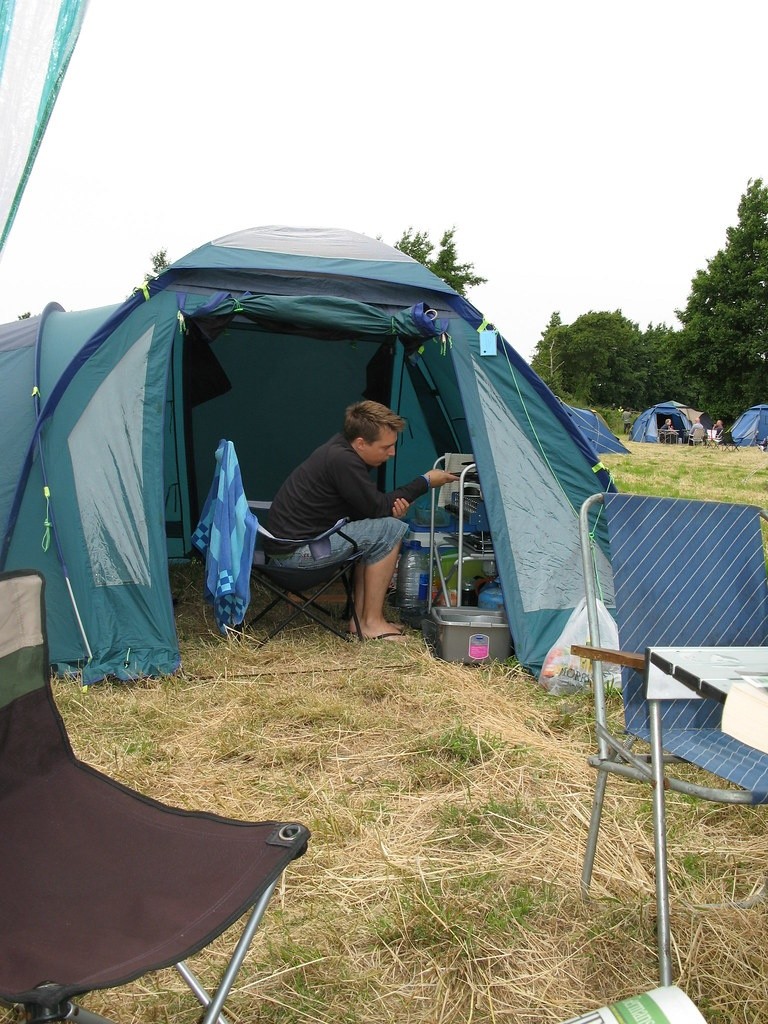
[344,621,405,644]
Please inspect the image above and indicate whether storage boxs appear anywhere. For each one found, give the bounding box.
[431,606,511,665]
[403,518,485,592]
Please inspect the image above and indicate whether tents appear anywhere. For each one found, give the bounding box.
[0,225,619,687]
[628,401,716,444]
[554,395,631,455]
[725,405,768,446]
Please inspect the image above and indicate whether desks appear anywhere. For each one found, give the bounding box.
[645,645,768,732]
[657,429,680,444]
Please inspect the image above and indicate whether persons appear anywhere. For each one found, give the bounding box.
[660,419,676,444]
[689,418,706,446]
[618,405,632,435]
[712,420,724,445]
[264,401,460,645]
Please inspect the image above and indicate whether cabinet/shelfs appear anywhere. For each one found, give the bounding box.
[426,454,495,615]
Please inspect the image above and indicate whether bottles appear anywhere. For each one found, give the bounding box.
[430,560,442,604]
[397,540,428,608]
[462,580,478,607]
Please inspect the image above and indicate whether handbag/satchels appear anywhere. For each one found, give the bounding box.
[537,596,622,697]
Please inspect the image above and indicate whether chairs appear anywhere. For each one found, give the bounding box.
[688,429,706,447]
[707,431,744,452]
[0,568,312,1024]
[217,439,366,648]
[569,492,768,989]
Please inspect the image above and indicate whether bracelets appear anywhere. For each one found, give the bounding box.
[421,475,431,487]
